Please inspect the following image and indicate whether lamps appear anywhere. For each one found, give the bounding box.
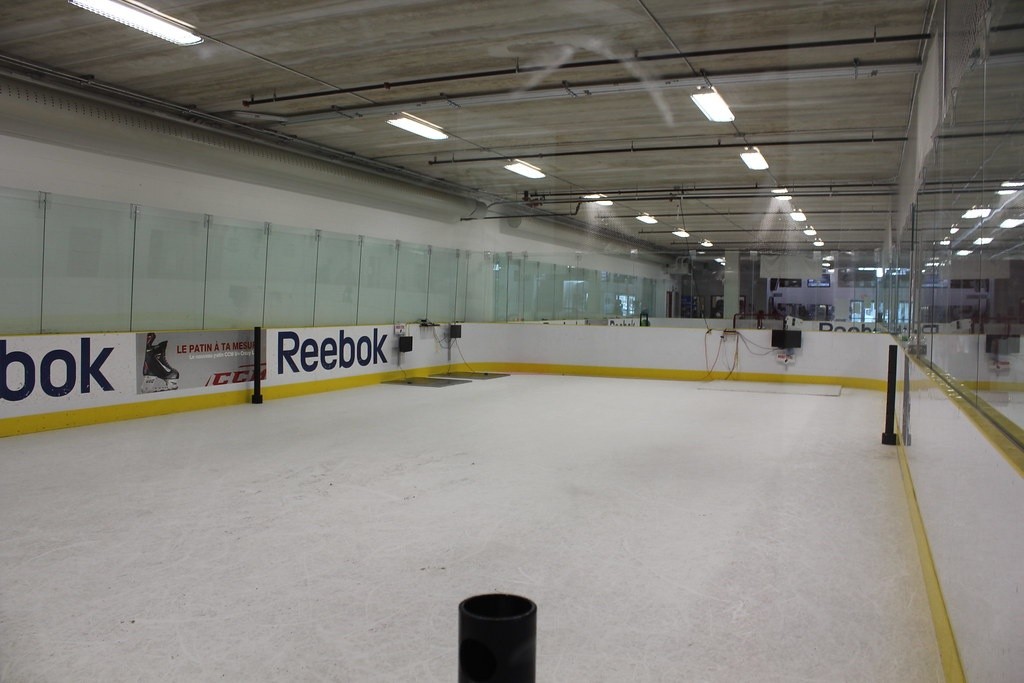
[772,187,825,247]
[66,0,204,46]
[504,158,546,179]
[691,83,736,123]
[739,144,770,171]
[584,193,713,248]
[387,112,450,141]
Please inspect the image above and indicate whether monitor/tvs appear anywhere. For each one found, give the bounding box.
[985,334,1020,355]
[771,330,802,348]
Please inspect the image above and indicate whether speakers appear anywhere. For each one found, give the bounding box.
[399,337,413,351]
[450,325,462,338]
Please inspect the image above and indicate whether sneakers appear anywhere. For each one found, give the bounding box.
[141,333,179,393]
